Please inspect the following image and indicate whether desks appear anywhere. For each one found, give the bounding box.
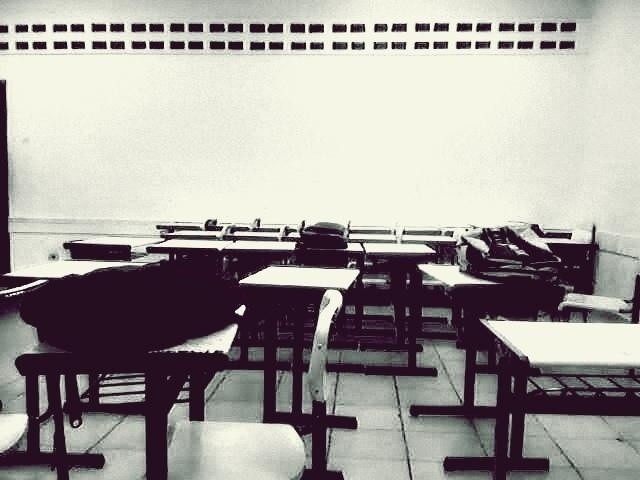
[1,220,638,480]
